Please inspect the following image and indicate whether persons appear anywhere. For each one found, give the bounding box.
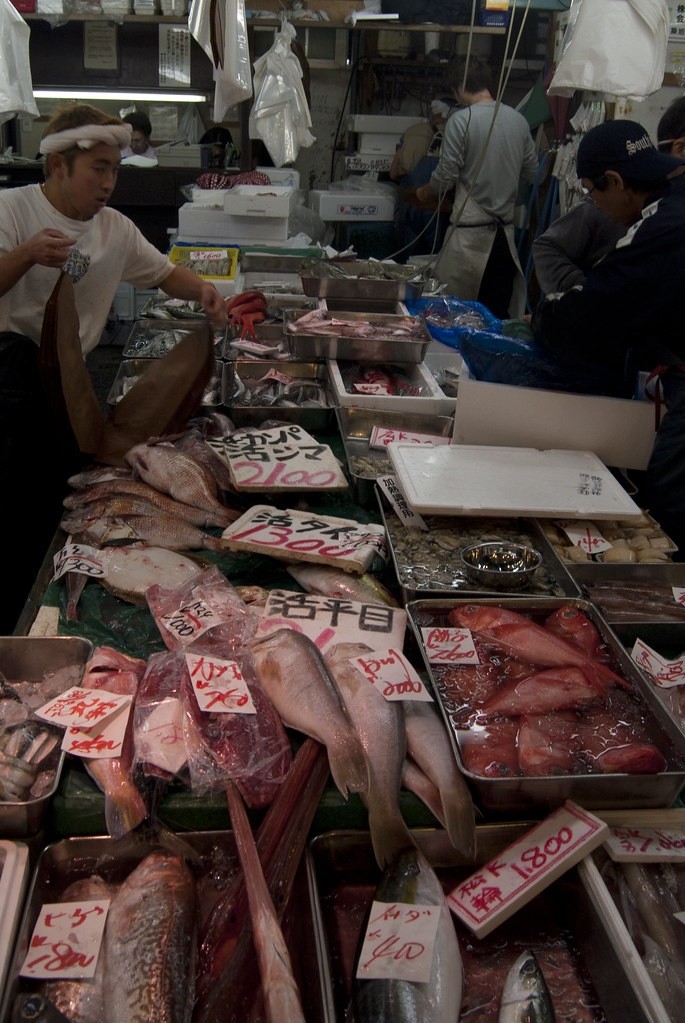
[523,97,685,564]
[417,54,551,320]
[390,97,461,251]
[0,105,228,628]
[120,112,156,159]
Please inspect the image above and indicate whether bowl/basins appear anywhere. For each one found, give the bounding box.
[460,541,542,589]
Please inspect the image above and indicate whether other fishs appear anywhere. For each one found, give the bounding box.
[103,249,488,407]
[0,440,685,1023]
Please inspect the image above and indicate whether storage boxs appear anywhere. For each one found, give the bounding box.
[1,114,685,1023]
